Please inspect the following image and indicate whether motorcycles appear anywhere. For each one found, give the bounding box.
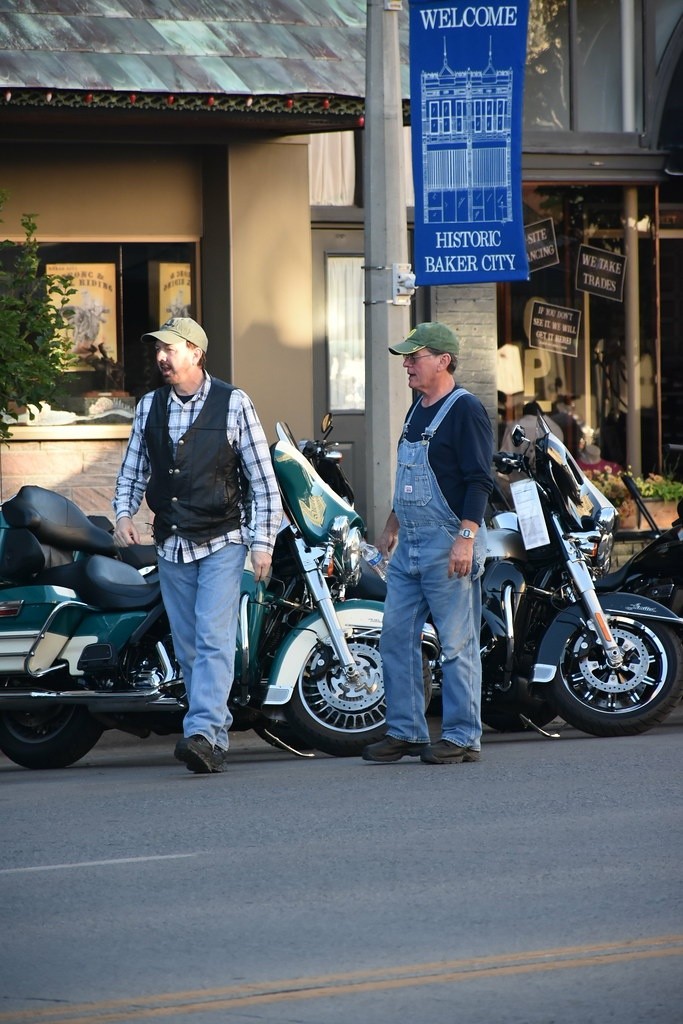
[0,400,682,771]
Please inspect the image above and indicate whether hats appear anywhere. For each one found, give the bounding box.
[388,322,459,356]
[141,317,208,354]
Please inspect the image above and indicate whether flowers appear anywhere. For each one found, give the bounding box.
[583,465,683,526]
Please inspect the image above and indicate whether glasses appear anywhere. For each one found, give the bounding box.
[402,352,442,364]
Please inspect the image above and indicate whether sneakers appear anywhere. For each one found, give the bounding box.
[420,738,480,764]
[173,733,213,774]
[186,745,225,772]
[362,734,431,762]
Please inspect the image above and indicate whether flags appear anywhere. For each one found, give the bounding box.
[409,0,530,286]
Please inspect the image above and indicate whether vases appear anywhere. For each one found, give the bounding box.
[611,497,683,529]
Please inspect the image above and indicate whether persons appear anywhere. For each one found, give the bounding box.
[575,445,621,477]
[499,401,584,455]
[110,318,284,778]
[359,322,492,764]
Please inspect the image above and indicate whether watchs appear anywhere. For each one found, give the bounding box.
[457,528,475,539]
